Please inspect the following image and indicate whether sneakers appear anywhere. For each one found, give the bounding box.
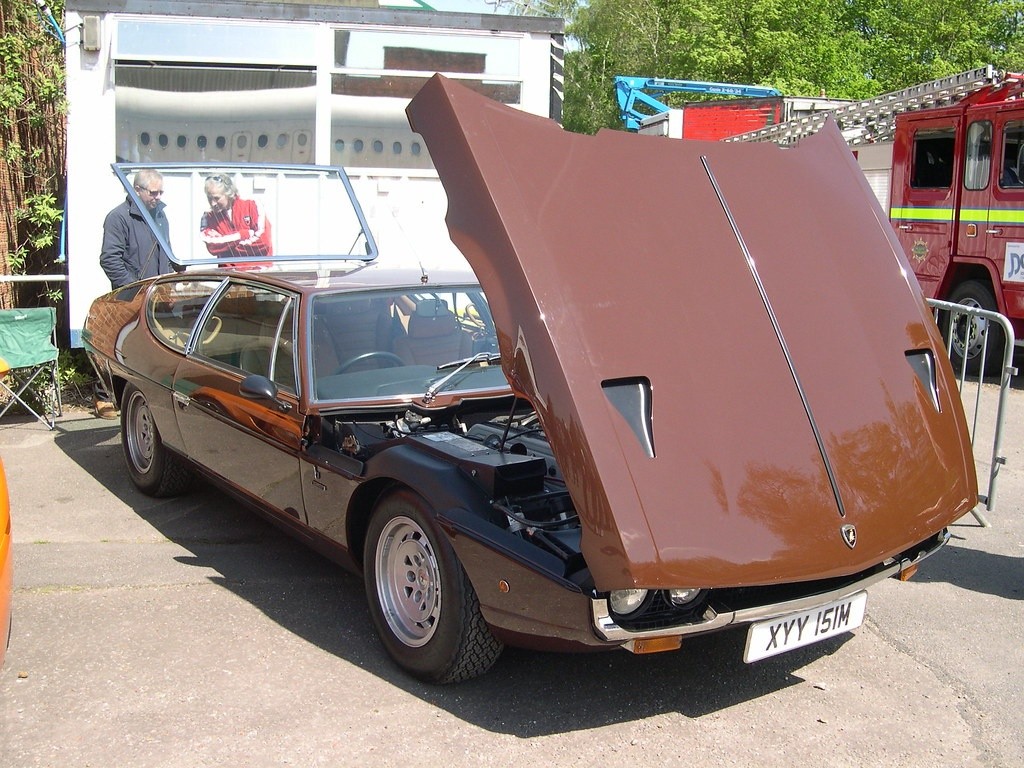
[92,393,117,420]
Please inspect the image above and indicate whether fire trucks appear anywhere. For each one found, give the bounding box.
[611,63,1024,379]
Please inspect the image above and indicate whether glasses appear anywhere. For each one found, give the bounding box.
[205,176,227,185]
[139,185,164,197]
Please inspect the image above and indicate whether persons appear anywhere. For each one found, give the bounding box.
[200,174,272,266]
[93,169,174,420]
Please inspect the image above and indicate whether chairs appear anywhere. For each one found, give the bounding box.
[238,313,295,390]
[393,312,472,369]
[0,306,63,432]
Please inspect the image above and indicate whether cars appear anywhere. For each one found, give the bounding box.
[81,72,981,684]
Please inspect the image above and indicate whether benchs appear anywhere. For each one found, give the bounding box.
[195,290,381,370]
